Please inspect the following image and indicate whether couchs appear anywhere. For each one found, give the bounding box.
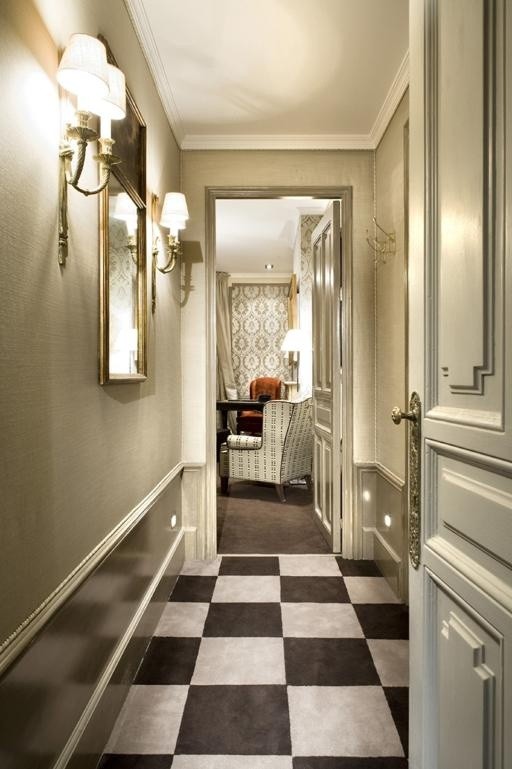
[218,397,315,501]
[236,376,280,436]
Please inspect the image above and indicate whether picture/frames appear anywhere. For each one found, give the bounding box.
[97,34,150,387]
[285,273,298,365]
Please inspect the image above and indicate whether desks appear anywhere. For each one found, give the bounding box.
[217,397,267,436]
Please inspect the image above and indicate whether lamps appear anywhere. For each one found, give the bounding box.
[282,327,301,364]
[51,31,127,265]
[151,185,188,276]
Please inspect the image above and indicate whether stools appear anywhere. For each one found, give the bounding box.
[216,428,230,462]
